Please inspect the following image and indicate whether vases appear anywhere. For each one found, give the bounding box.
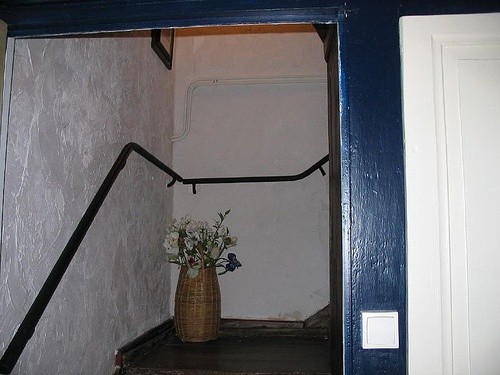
[174,263,222,344]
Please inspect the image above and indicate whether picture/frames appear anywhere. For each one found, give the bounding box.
[150,27,176,71]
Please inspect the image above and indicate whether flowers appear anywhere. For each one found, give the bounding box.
[162,210,242,276]
